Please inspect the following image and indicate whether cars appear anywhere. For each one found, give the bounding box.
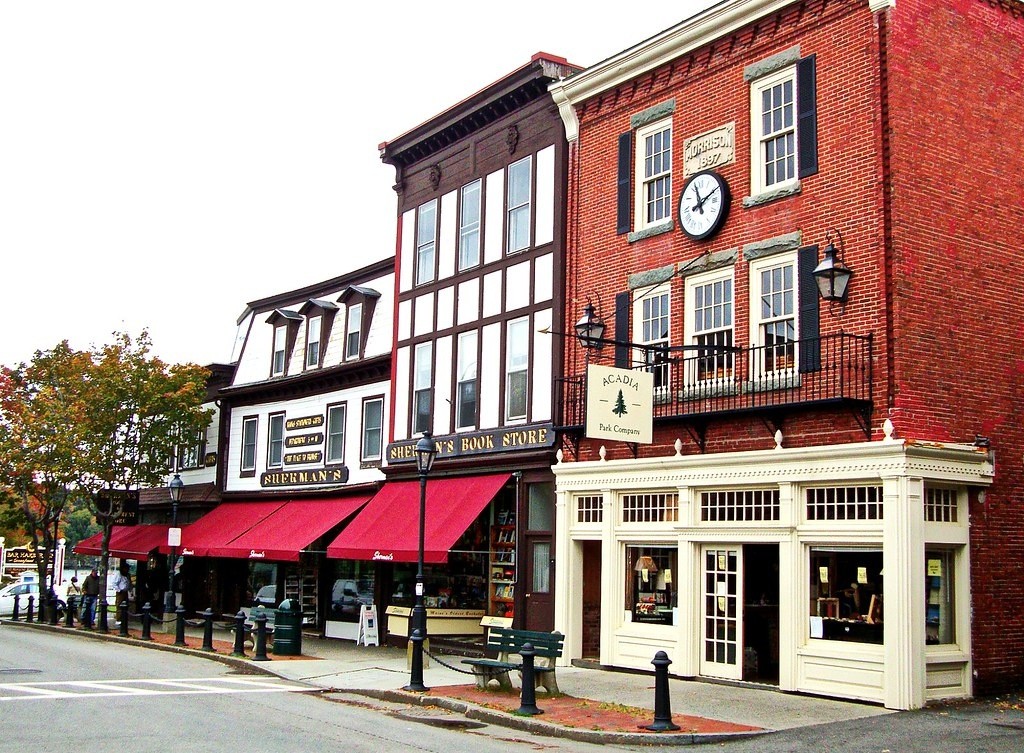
[0,581,70,619]
[330,572,487,623]
[251,584,277,609]
[51,569,125,613]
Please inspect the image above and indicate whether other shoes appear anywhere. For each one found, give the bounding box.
[115,621,121,625]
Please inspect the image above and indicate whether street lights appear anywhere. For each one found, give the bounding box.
[403,428,440,693]
[164,471,185,613]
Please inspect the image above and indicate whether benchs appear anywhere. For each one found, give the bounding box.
[461,626,565,698]
[239,607,279,645]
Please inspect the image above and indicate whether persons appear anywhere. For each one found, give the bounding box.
[111,564,133,625]
[67,578,81,597]
[77,567,100,629]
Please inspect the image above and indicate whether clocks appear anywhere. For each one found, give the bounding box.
[677,171,731,241]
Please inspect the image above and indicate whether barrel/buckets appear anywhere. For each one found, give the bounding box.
[273,610,303,655]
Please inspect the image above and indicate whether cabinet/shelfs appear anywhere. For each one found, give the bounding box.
[489,523,519,628]
[284,566,319,629]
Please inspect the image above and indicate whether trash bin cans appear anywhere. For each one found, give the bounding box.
[273,599,302,656]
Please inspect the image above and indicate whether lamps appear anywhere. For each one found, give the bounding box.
[573,290,607,350]
[811,226,852,318]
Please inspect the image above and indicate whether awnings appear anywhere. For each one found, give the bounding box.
[207,494,376,562]
[73,523,189,562]
[158,498,290,557]
[325,472,522,564]
